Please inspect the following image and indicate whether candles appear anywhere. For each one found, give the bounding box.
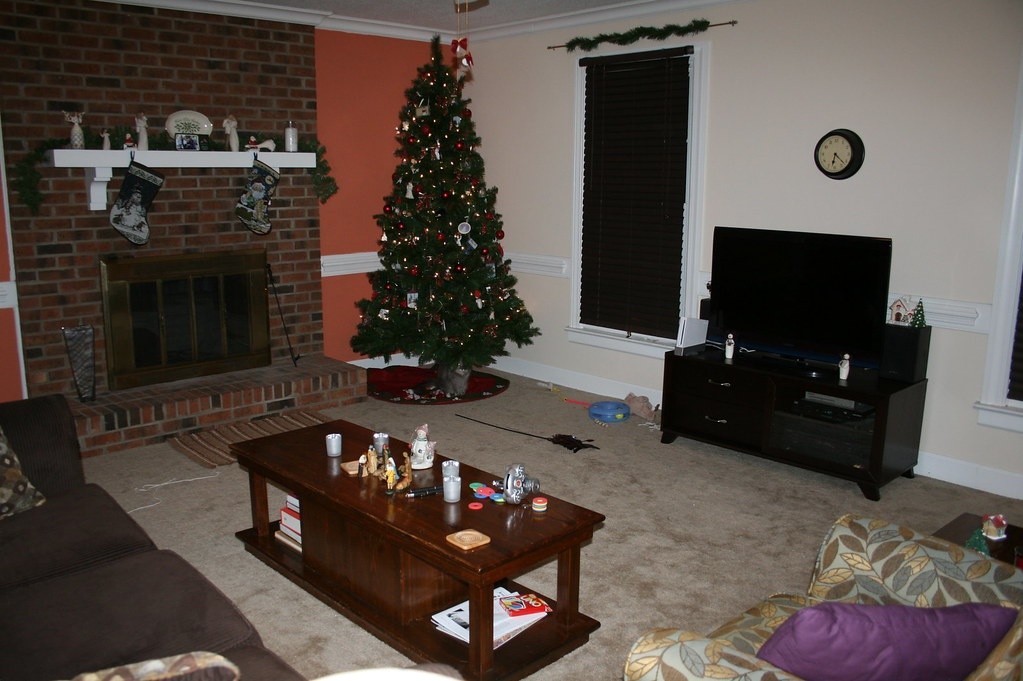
[441,460,462,502]
[372,433,390,456]
[326,434,342,457]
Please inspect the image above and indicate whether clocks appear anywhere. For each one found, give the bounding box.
[812,128,865,181]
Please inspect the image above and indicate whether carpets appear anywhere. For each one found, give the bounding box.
[167,408,333,470]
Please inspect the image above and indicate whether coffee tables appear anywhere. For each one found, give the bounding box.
[932,512,1022,565]
[226,418,606,681]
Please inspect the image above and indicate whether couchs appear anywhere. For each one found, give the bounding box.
[623,512,1023,681]
[0,393,465,681]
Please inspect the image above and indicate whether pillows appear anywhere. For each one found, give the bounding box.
[755,602,1020,681]
[56,648,241,681]
[0,426,47,523]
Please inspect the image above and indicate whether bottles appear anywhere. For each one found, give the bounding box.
[284,120,298,153]
[726,334,734,359]
[839,354,850,380]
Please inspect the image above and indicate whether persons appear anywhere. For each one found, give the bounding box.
[64,113,259,152]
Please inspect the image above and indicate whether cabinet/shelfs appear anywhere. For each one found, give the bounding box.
[659,342,928,502]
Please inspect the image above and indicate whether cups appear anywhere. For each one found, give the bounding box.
[442,461,459,477]
[326,433,342,457]
[443,477,461,503]
[373,432,389,457]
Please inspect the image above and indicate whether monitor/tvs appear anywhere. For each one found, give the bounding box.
[705,226,892,369]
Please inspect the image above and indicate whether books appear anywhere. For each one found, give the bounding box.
[430,586,547,650]
[274,494,302,554]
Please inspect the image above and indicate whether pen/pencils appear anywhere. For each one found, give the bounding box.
[406,486,443,497]
